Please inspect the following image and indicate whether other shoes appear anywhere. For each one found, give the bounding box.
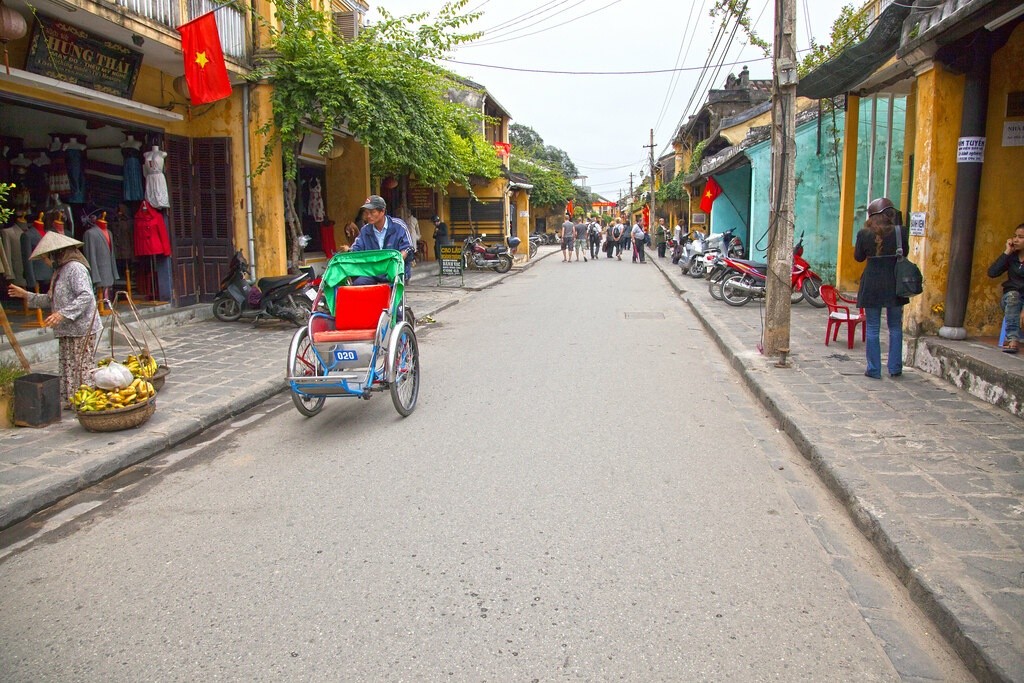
[617,255,622,261]
[1002,341,1019,352]
[632,261,637,263]
[865,371,873,377]
[640,260,647,264]
[891,372,902,377]
[595,255,599,259]
[591,256,594,260]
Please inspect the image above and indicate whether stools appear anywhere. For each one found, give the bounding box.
[999,315,1024,349]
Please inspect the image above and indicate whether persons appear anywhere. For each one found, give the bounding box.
[283,173,336,259]
[986,223,1024,353]
[701,226,708,235]
[853,196,910,380]
[354,207,369,232]
[430,215,449,276]
[339,220,360,253]
[560,214,647,265]
[347,194,416,324]
[407,207,422,268]
[6,230,105,411]
[670,218,691,258]
[0,131,174,289]
[656,217,670,258]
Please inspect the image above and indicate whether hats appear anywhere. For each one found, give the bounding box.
[362,195,386,211]
[430,215,440,222]
[29,230,85,260]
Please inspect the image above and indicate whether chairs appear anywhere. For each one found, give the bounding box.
[819,283,867,351]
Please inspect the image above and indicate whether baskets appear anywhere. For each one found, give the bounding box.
[132,364,171,394]
[76,388,157,433]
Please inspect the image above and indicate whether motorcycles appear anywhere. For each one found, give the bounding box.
[463,233,521,273]
[213,248,317,327]
[668,230,827,309]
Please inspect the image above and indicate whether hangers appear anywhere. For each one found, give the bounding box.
[307,175,321,184]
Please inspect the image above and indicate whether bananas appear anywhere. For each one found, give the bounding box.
[68,351,159,412]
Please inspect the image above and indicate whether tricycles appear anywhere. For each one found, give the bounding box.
[283,250,419,418]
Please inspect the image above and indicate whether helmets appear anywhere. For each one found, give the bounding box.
[868,198,894,216]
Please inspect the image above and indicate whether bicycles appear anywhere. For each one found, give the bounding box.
[529,233,554,259]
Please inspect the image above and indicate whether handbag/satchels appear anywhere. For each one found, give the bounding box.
[894,257,923,298]
[643,233,650,243]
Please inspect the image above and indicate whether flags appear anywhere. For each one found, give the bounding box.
[698,175,723,214]
[494,141,512,170]
[566,198,574,219]
[174,10,232,107]
[642,206,650,231]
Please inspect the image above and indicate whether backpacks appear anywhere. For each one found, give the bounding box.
[613,224,621,237]
[589,222,598,241]
